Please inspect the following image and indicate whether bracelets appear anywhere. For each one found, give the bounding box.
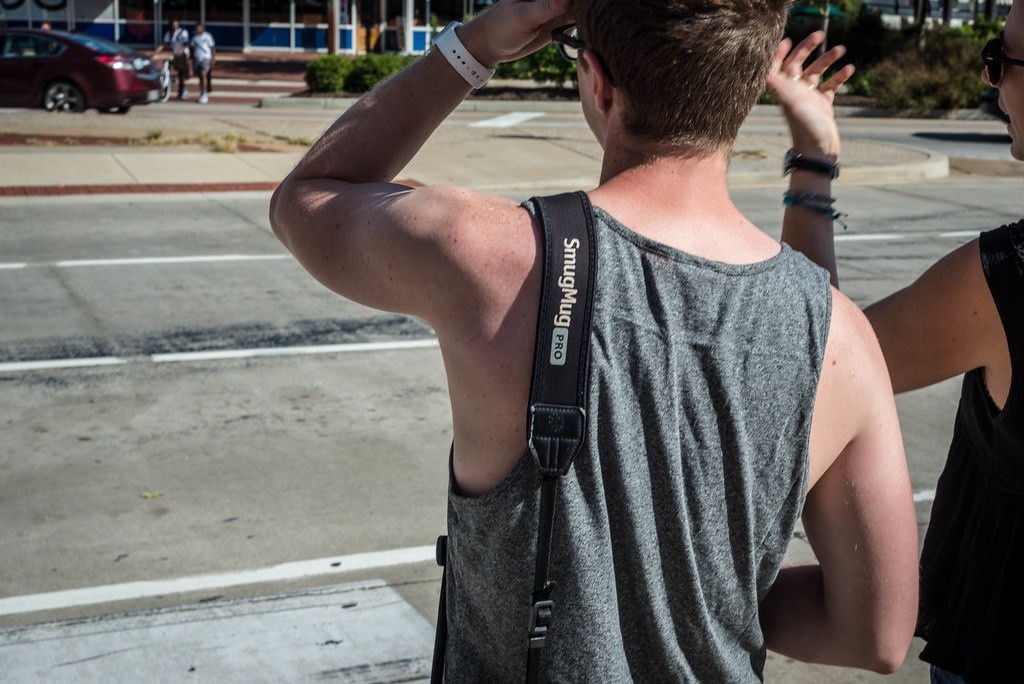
[783,190,848,231]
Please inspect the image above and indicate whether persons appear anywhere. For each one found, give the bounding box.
[189,24,216,103]
[767,1,1024,683]
[268,1,920,683]
[33,23,56,53]
[155,18,190,100]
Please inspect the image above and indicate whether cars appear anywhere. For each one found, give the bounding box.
[0,28,163,115]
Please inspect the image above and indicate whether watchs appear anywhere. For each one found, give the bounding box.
[431,20,497,90]
[783,148,839,181]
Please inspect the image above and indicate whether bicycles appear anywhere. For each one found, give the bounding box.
[152,56,176,103]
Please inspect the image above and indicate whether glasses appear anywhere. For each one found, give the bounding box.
[549,21,590,65]
[979,34,1024,89]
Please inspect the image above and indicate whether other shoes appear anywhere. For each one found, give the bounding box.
[198,92,209,103]
